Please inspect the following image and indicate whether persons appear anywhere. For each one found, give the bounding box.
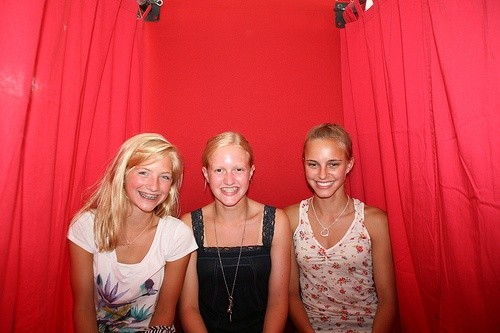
[64,132,199,333]
[179,130,293,333]
[281,120,397,333]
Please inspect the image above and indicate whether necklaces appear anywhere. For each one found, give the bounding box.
[213,198,248,323]
[310,193,350,237]
[121,211,156,248]
